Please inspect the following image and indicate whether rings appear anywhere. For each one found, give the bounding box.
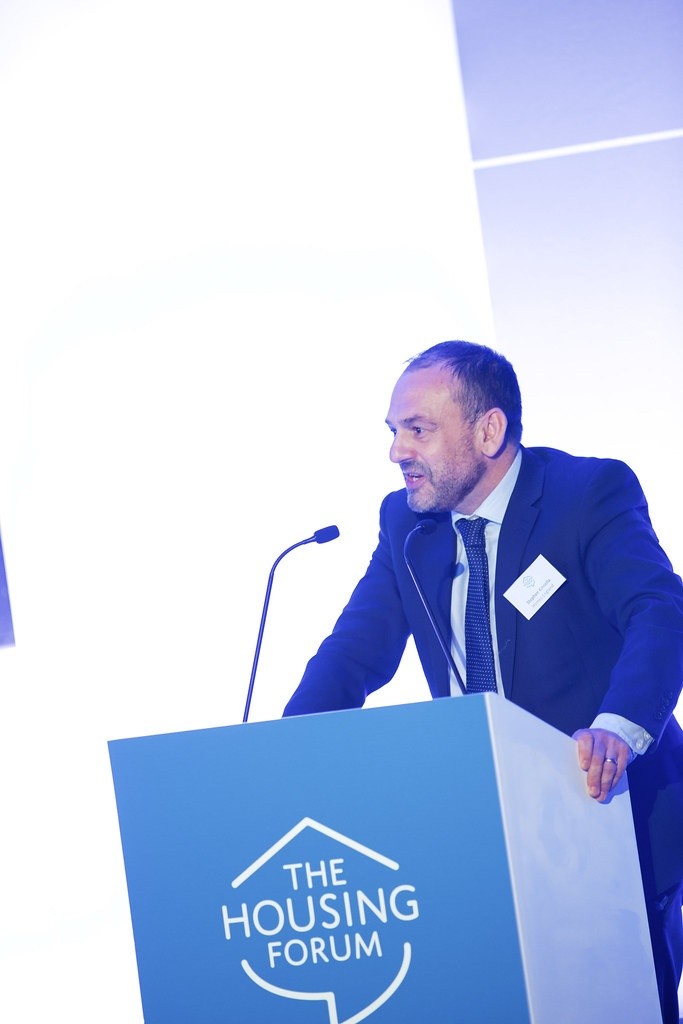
[604,757,618,764]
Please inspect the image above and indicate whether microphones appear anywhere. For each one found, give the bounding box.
[404,519,468,696]
[243,524,341,723]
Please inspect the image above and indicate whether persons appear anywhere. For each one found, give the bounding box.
[280,340,683,1024]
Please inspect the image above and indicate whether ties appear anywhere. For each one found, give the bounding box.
[455,517,498,694]
